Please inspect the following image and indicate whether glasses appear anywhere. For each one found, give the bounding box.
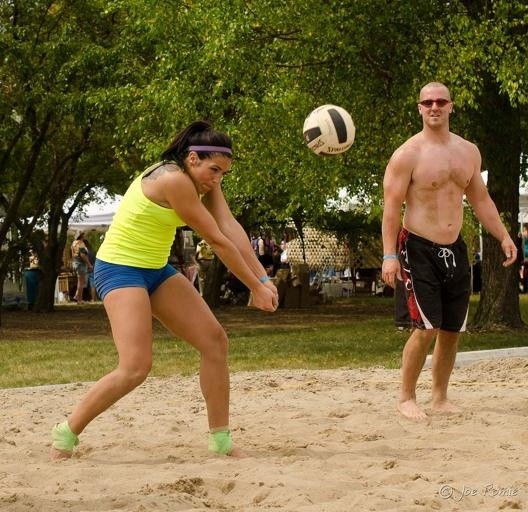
[420,98,451,109]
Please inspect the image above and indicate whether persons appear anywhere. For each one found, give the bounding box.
[71,219,528,303]
[48,122,283,466]
[381,82,518,422]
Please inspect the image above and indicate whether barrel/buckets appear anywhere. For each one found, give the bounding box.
[24,267,41,310]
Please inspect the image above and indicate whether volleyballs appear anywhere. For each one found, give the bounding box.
[302,104,356,158]
